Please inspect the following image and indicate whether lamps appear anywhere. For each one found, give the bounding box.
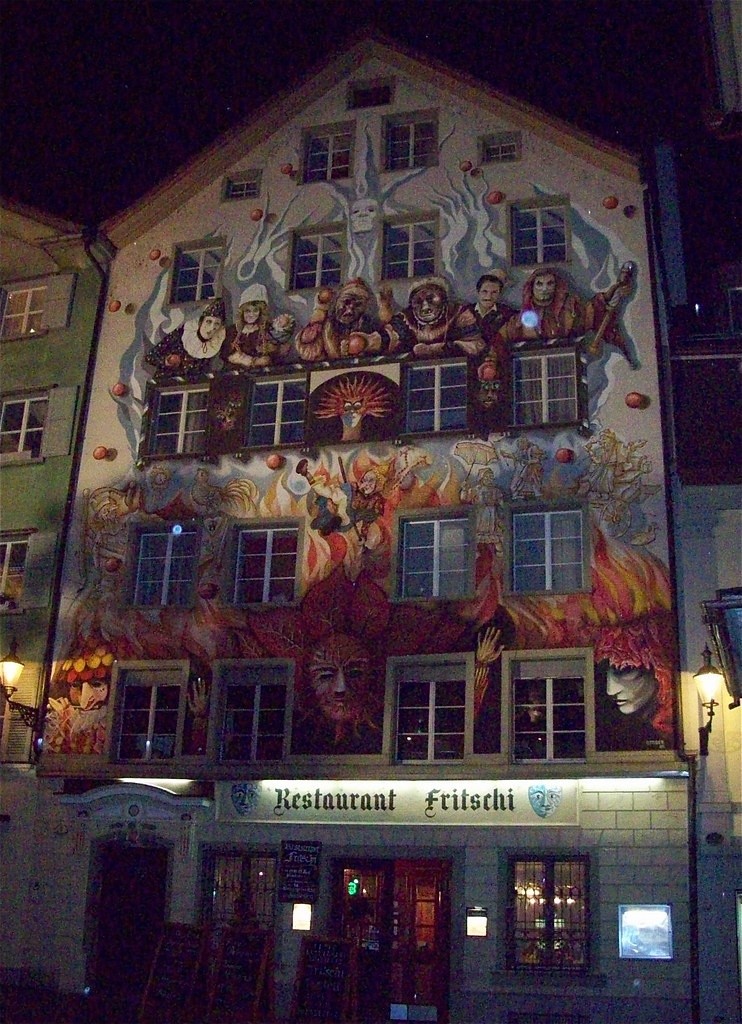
[692,641,724,757]
[0,636,38,731]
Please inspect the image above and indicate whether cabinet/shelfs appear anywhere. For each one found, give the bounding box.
[134,922,359,1024]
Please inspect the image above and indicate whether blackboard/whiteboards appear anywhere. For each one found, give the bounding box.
[207,925,272,1021]
[140,921,211,1016]
[290,934,356,1024]
